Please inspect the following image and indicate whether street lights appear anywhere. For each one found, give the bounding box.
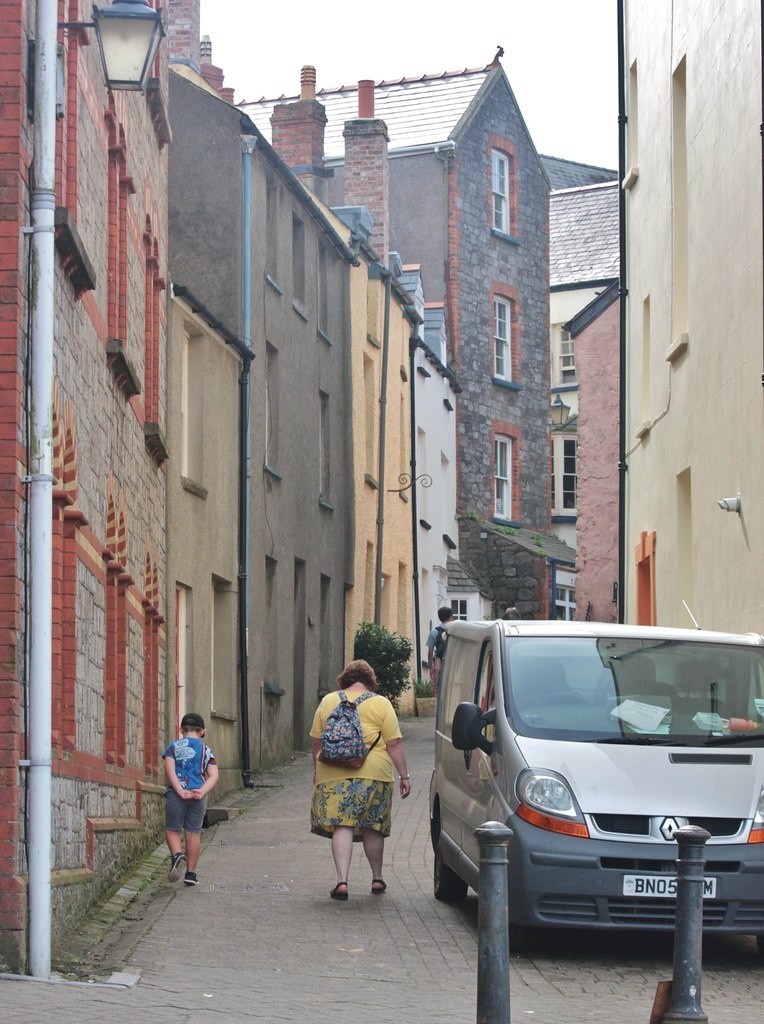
[371,251,408,628]
[238,133,261,651]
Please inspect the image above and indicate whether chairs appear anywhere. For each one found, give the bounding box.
[599,654,677,703]
[520,655,569,706]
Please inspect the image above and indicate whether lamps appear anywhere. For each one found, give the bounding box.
[58,0,167,91]
[546,393,579,431]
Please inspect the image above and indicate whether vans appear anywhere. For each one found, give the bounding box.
[423,616,764,958]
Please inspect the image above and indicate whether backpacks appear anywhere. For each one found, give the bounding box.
[435,626,449,659]
[318,691,381,768]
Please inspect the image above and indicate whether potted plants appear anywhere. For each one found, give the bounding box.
[411,676,438,717]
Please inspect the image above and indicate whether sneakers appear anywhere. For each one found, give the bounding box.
[168,852,187,882]
[184,871,199,885]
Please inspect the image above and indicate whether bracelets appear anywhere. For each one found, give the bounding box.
[399,774,410,779]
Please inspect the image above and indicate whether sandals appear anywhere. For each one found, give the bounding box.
[372,879,386,893]
[330,882,348,899]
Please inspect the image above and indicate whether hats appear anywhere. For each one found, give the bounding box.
[181,713,205,738]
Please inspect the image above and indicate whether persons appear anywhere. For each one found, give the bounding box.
[161,712,218,884]
[310,659,411,899]
[426,607,454,694]
